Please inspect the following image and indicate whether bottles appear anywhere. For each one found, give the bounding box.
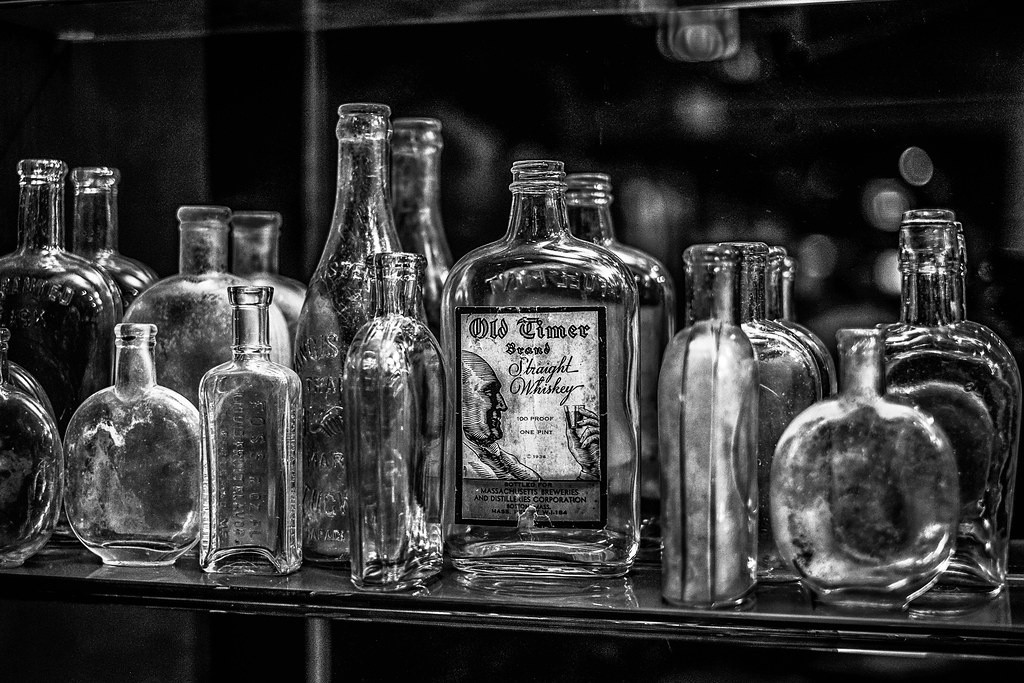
[62,324,200,568]
[568,174,673,554]
[0,342,65,571]
[121,207,292,557]
[767,248,837,394]
[873,209,1022,615]
[658,245,759,606]
[292,104,404,563]
[232,210,310,351]
[718,242,823,582]
[71,167,160,319]
[0,159,116,546]
[771,330,960,612]
[200,287,302,576]
[0,328,58,428]
[391,119,450,350]
[442,160,638,579]
[346,254,444,590]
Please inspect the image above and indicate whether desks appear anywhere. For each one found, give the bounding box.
[0,549,1024,683]
